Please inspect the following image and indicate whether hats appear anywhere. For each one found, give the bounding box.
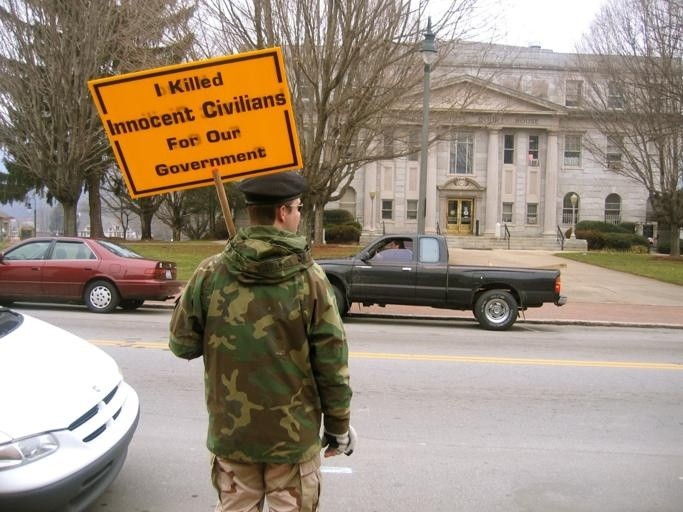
[239,171,304,208]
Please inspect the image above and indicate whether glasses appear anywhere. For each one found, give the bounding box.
[286,203,304,211]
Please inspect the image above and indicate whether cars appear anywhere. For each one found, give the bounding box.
[0,306,141,512]
[0,236,182,313]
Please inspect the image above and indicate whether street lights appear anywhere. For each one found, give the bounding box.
[570,194,578,239]
[417,16,439,234]
[368,189,376,235]
[27,196,37,238]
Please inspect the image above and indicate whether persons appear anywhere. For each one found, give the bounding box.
[376,240,410,262]
[168,171,358,512]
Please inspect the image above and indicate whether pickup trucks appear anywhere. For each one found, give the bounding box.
[313,233,567,330]
